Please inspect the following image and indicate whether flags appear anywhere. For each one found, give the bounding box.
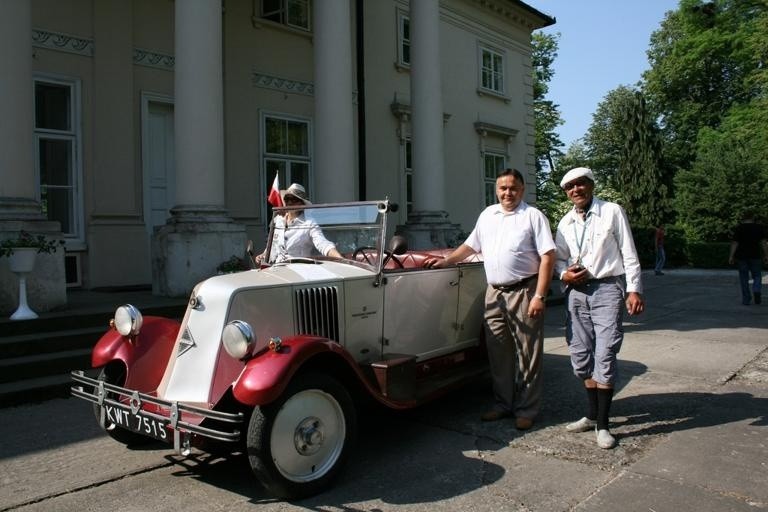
[267,172,281,207]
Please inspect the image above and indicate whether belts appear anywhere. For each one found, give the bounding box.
[492,274,538,291]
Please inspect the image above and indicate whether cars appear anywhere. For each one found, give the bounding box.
[71,194,486,500]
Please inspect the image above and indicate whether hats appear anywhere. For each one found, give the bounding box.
[560,167,595,190]
[279,183,313,207]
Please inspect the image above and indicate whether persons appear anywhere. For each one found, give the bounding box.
[553,167,644,449]
[421,166,556,432]
[652,218,665,276]
[254,183,347,263]
[728,210,767,304]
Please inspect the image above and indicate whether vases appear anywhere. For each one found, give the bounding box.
[2,247,41,320]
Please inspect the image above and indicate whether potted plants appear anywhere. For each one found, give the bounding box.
[216,254,250,275]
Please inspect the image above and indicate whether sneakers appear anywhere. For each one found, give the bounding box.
[566,416,598,433]
[480,409,506,421]
[515,416,534,431]
[595,422,617,449]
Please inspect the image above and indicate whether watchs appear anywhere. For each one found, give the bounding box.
[534,294,547,302]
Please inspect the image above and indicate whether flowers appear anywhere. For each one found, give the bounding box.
[0,230,66,257]
